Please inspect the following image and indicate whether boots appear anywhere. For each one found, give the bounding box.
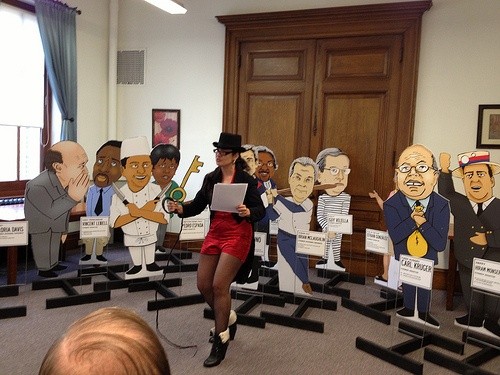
[203,327,230,368]
[209,310,237,343]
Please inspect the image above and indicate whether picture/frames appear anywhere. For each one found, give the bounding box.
[477,104,500,149]
[150,108,181,151]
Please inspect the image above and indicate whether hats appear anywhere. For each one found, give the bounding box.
[213,133,246,152]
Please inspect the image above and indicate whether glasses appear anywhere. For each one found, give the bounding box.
[213,149,233,155]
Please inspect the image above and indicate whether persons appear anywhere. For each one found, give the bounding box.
[39,307,171,375]
[168,132,266,366]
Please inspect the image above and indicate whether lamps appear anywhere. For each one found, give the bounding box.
[145,0,189,16]
